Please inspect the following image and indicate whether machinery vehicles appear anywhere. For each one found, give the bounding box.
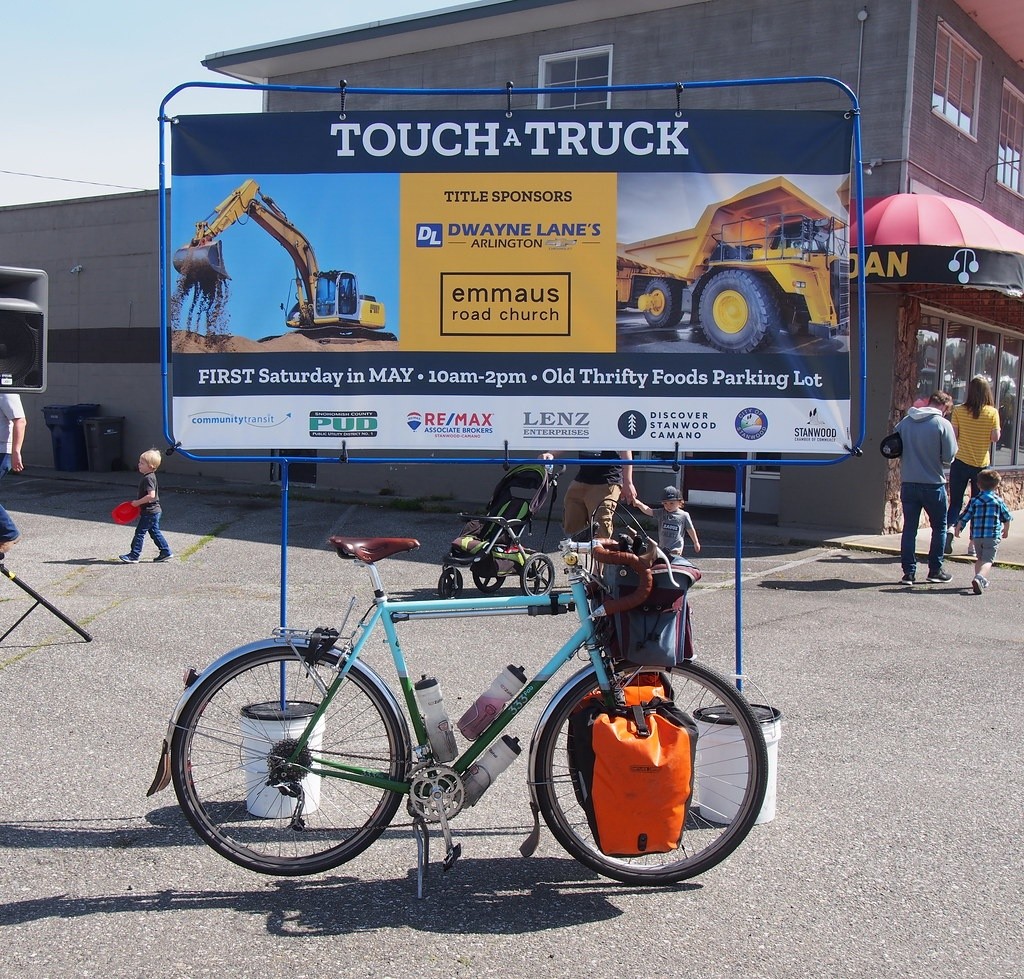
[173,179,397,344]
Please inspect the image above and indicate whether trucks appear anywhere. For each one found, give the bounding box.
[617,176,850,354]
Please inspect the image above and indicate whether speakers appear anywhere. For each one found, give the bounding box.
[0,266,49,395]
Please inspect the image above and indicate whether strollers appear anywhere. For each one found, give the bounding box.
[437,464,566,600]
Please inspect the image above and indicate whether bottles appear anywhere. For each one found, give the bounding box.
[450,736,522,810]
[457,665,528,739]
[415,674,458,762]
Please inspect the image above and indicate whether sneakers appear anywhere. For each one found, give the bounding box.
[902,575,915,584]
[119,554,139,563]
[926,568,954,582]
[153,550,174,562]
[972,573,990,595]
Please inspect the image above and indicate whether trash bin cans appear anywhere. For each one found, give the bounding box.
[82,416,124,472]
[41,403,100,472]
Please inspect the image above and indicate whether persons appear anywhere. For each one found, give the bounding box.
[537,450,637,581]
[119,450,174,563]
[0,393,27,553]
[893,390,959,585]
[944,376,1000,556]
[954,470,1013,595]
[633,486,700,556]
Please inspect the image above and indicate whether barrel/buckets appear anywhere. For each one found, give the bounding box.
[694,675,782,825]
[239,699,326,819]
[112,503,140,525]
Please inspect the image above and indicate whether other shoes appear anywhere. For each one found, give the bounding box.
[968,543,976,556]
[0,534,20,553]
[944,524,955,555]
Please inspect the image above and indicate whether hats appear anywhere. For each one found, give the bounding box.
[657,485,682,502]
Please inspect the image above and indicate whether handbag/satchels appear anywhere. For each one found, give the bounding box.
[595,547,701,666]
[569,673,699,855]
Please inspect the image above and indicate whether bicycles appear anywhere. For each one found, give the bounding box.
[145,497,769,899]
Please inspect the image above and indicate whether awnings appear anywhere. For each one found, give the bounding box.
[850,194,1024,305]
